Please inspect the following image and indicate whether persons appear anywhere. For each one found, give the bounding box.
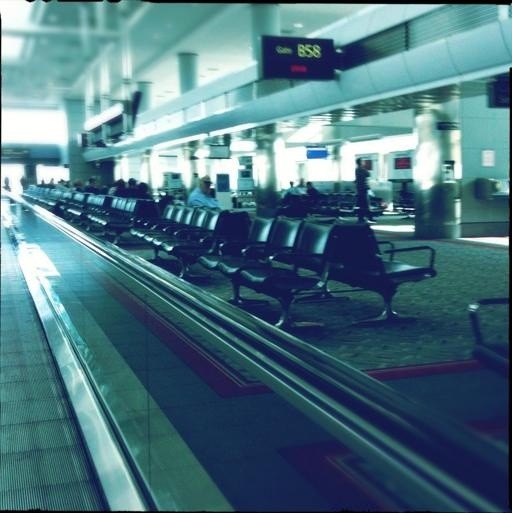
[306,180,318,192]
[398,181,411,197]
[20,175,155,200]
[354,156,375,223]
[186,174,221,211]
[287,180,298,192]
[189,171,201,193]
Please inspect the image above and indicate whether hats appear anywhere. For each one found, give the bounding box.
[201,175,212,184]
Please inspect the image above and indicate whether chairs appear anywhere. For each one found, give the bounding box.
[469,297,511,379]
[130,204,435,330]
[23,184,175,245]
[271,189,386,220]
